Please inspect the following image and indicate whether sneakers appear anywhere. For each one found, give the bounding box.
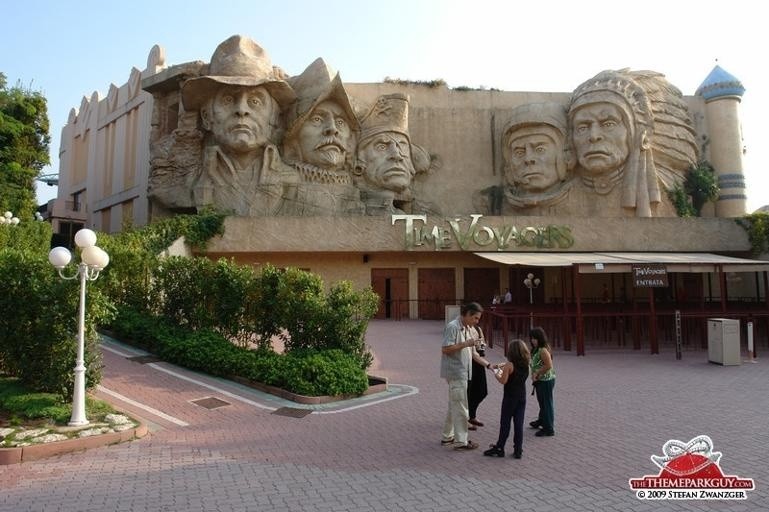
[484,446,523,458]
[441,438,480,450]
[467,420,484,430]
[529,420,554,436]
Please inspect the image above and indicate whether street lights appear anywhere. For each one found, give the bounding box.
[524,273,541,329]
[49,228,109,426]
[32,211,43,222]
[0,211,19,225]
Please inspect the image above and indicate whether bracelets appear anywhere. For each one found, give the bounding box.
[486,363,492,368]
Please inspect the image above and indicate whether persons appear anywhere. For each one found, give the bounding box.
[352,93,431,216]
[467,317,488,431]
[490,289,503,305]
[527,326,557,436]
[568,67,700,217]
[502,288,512,304]
[482,338,530,458]
[182,35,297,218]
[283,58,365,218]
[502,102,575,216]
[440,302,496,451]
